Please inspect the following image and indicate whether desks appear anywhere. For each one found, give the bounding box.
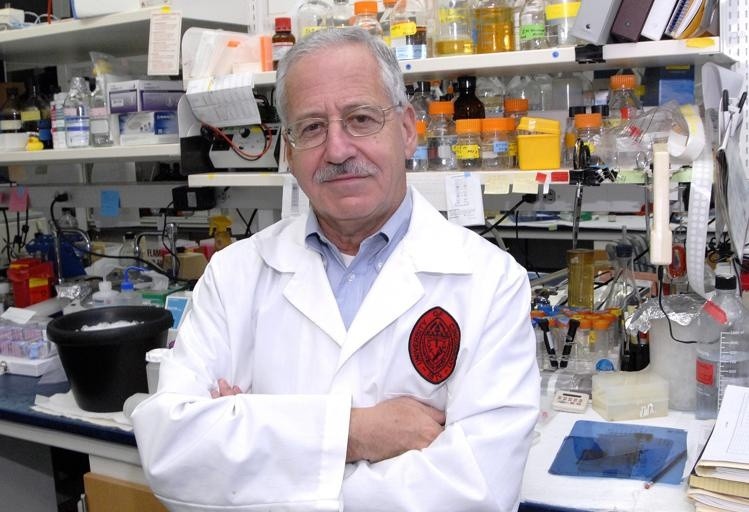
[1,370,152,512]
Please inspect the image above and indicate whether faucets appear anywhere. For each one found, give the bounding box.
[133,222,176,280]
[53,227,93,281]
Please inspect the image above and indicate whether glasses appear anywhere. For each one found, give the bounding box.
[282,101,407,153]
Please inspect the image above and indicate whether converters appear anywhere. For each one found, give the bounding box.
[171,186,217,212]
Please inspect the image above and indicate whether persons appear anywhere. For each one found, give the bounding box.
[128,24,543,512]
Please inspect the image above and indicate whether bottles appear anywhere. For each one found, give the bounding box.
[145,347,171,397]
[567,248,597,311]
[119,232,145,281]
[20,83,49,127]
[401,67,648,173]
[297,0,581,60]
[61,75,116,149]
[530,301,622,371]
[601,244,643,309]
[1,87,26,134]
[90,280,145,307]
[270,16,297,72]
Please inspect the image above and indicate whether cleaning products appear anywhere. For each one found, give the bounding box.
[91,265,119,308]
[63,75,112,148]
[115,266,145,306]
[206,215,233,251]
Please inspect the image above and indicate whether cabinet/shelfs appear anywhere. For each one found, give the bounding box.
[186,35,724,188]
[77,472,168,512]
[1,9,252,164]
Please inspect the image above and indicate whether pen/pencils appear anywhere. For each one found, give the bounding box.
[644,450,687,488]
